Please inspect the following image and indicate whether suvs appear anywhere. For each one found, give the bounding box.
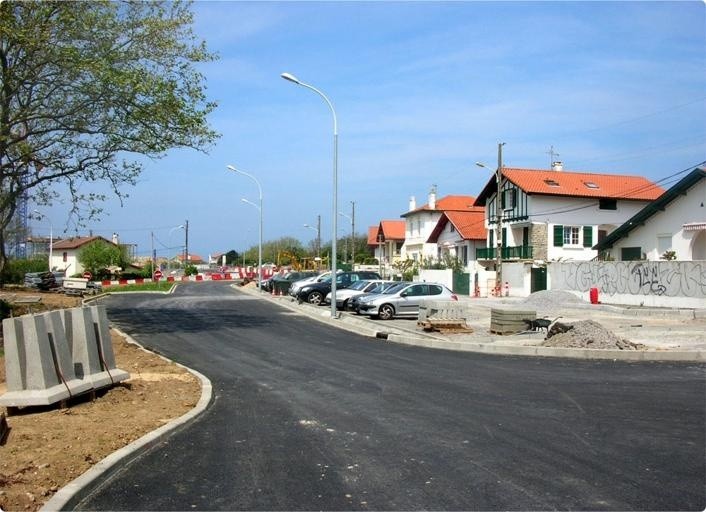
[297,270,382,305]
[256,271,321,296]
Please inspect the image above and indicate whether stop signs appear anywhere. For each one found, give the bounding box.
[82,271,91,280]
[155,270,162,279]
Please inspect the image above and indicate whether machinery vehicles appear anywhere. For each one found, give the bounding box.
[273,248,322,271]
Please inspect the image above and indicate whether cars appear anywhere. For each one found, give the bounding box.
[289,271,333,296]
[325,280,459,320]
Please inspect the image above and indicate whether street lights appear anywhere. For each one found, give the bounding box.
[226,165,262,291]
[475,161,501,288]
[33,210,53,258]
[303,225,320,258]
[167,226,185,271]
[339,212,355,267]
[282,72,339,319]
